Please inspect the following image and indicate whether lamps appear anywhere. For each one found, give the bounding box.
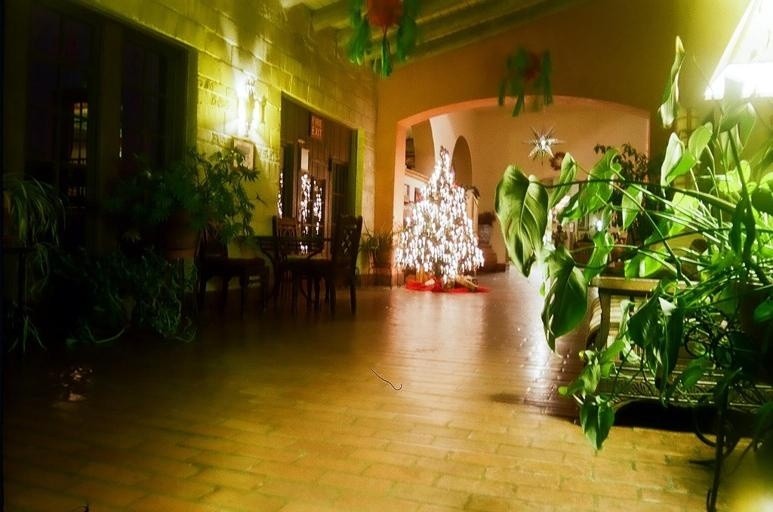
[247,76,267,122]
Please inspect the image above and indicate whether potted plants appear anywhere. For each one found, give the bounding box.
[103,142,257,309]
[357,226,408,268]
[0,171,75,249]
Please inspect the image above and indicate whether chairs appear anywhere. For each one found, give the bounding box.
[198,214,363,322]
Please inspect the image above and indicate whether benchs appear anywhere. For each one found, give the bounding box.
[586,235,773,424]
